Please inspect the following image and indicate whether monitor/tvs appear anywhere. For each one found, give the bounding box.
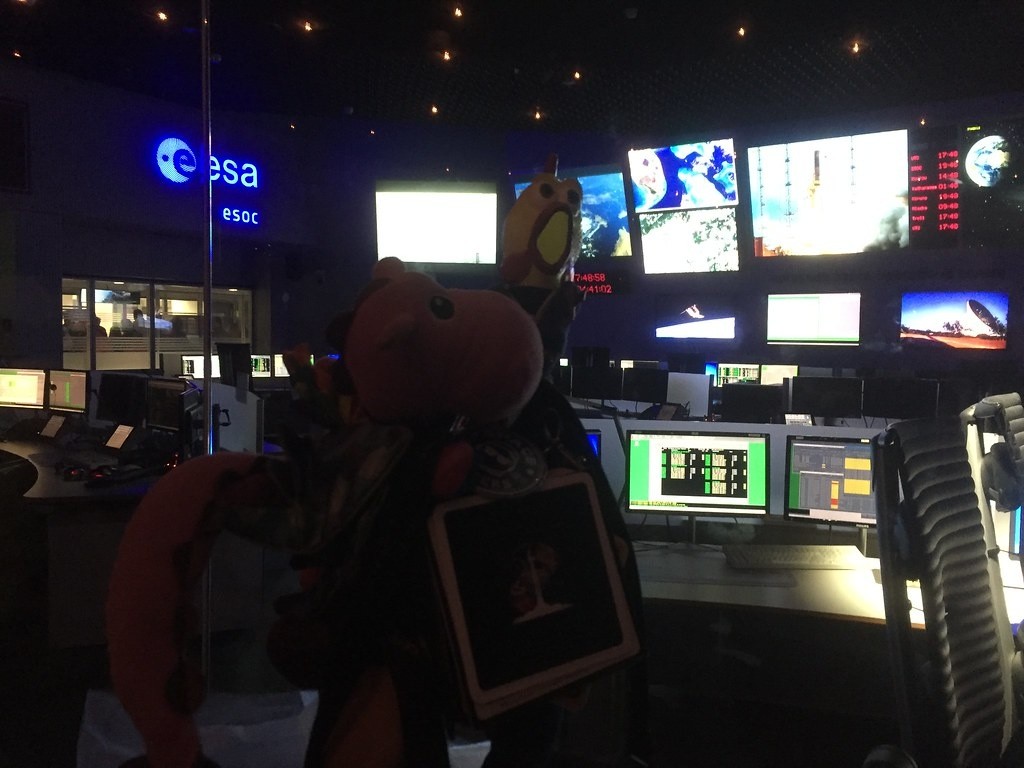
[369,114,1024,529]
[0,353,314,457]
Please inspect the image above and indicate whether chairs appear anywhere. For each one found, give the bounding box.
[863,415,1016,768]
[960,392,1024,665]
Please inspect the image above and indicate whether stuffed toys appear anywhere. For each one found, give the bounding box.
[106,258,544,768]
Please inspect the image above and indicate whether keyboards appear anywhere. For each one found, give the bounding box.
[722,543,869,570]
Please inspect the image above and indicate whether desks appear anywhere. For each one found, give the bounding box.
[0,440,265,651]
[631,540,929,661]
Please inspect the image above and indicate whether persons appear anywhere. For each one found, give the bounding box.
[94,309,146,336]
